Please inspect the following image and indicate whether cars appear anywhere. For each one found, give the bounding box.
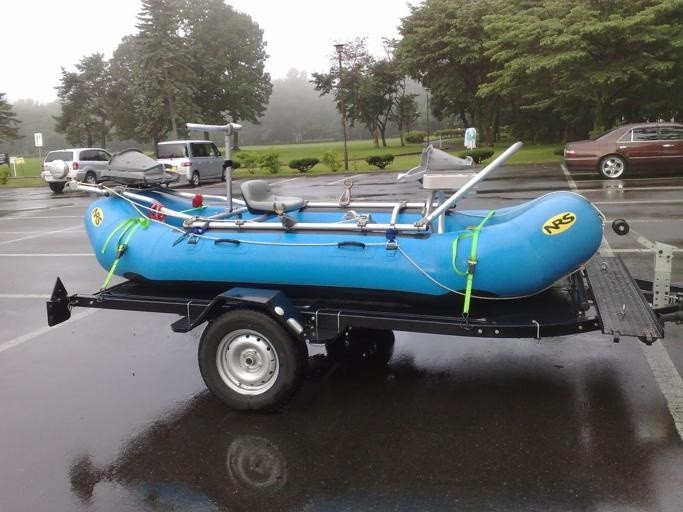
[563,122,683,180]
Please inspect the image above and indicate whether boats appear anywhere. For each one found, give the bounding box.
[61,122,605,306]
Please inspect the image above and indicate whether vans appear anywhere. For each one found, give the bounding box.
[156,139,226,186]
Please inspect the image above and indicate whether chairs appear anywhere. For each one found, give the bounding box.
[238,179,307,216]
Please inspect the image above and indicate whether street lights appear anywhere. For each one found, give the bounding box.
[332,44,349,170]
[424,88,431,146]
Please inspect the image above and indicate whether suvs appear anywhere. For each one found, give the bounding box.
[40,147,113,192]
